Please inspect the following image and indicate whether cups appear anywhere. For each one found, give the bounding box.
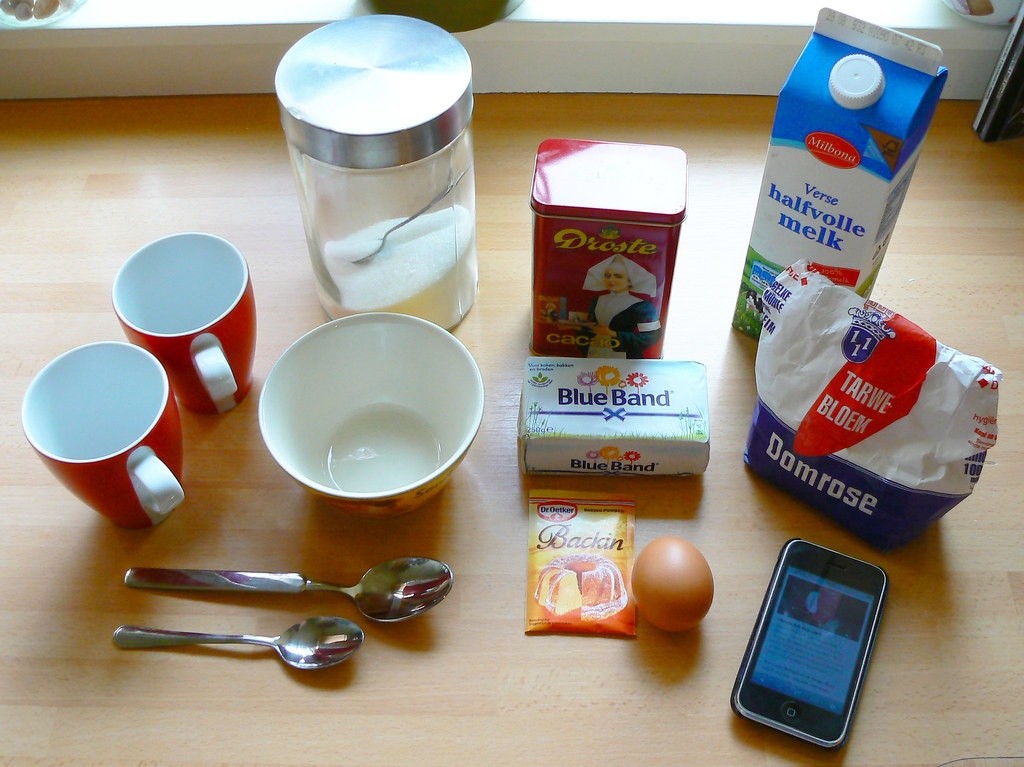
[22,340,186,531]
[111,231,257,414]
[274,17,480,330]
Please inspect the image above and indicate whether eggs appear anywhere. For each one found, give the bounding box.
[631,535,714,632]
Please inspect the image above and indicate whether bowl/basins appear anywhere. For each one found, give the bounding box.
[258,311,485,520]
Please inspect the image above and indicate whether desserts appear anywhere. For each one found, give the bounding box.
[535,555,627,621]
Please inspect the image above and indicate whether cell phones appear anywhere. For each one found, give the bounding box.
[730,538,889,751]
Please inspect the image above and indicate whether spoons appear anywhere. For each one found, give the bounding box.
[125,558,454,622]
[350,165,470,265]
[112,617,365,670]
